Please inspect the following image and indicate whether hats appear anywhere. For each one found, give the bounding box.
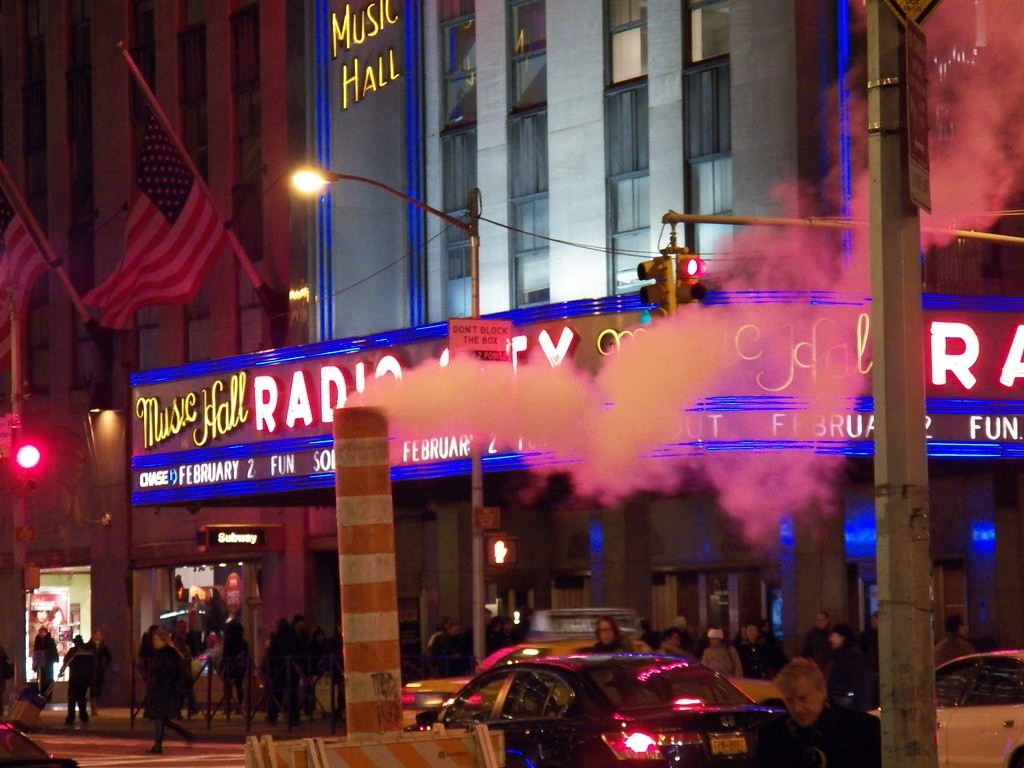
[673,616,686,626]
[707,628,723,639]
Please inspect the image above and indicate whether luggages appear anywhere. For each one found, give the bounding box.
[9,674,60,732]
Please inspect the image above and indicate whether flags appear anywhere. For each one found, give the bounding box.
[77,100,229,330]
[1,154,58,334]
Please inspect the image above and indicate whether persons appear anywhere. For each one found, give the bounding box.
[933,612,978,668]
[625,611,879,710]
[29,597,69,659]
[587,617,634,654]
[32,626,60,704]
[422,605,523,676]
[135,606,347,753]
[56,634,95,723]
[84,630,112,709]
[754,657,883,768]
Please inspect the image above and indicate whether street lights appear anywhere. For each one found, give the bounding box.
[287,165,484,664]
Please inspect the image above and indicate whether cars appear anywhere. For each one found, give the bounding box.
[401,605,784,732]
[403,654,809,768]
[868,648,1024,768]
[297,634,347,723]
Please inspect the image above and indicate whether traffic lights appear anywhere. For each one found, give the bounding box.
[637,253,679,320]
[675,253,706,305]
[11,434,50,478]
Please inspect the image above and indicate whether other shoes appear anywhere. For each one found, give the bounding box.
[144,748,161,755]
